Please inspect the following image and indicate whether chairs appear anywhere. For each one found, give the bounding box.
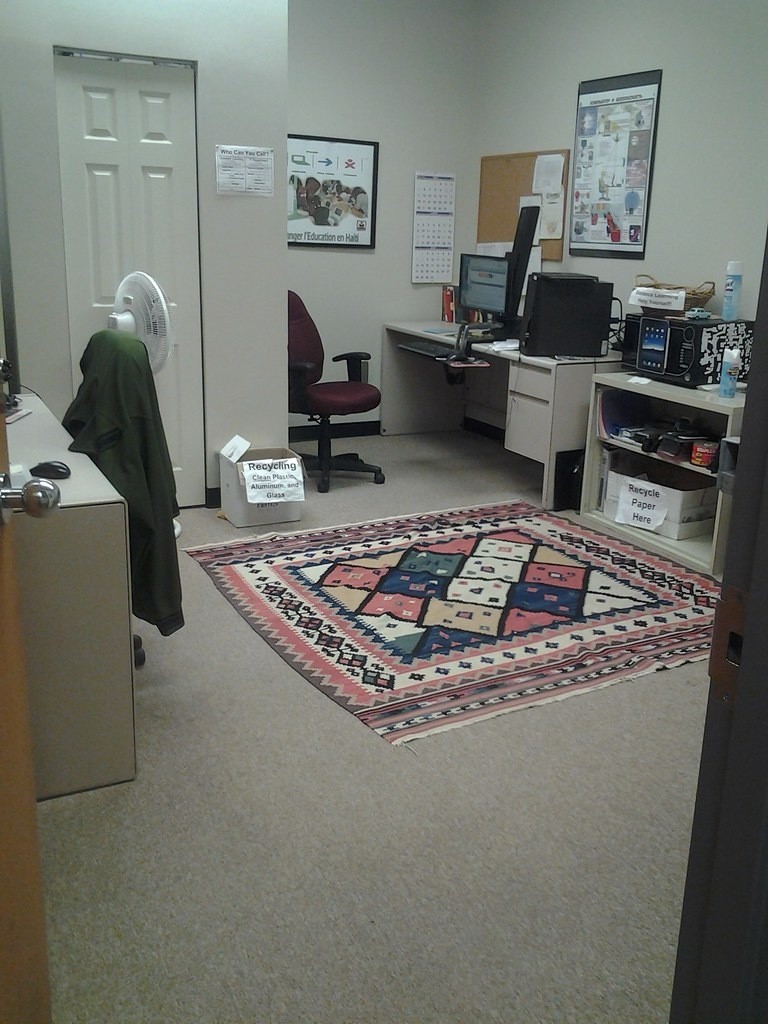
[288,289,386,494]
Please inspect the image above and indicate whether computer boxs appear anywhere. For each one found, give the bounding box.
[518,272,614,357]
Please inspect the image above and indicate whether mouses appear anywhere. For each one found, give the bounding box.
[447,353,469,362]
[29,461,71,479]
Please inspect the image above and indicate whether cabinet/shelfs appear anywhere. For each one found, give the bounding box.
[5,390,137,802]
[580,372,746,576]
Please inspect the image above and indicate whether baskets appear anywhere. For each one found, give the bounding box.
[633,274,715,318]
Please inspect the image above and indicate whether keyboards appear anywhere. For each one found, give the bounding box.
[398,342,454,357]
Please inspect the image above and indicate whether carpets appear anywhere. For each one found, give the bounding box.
[179,499,723,746]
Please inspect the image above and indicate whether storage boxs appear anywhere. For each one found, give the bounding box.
[106,271,173,377]
[603,468,718,541]
[219,435,310,528]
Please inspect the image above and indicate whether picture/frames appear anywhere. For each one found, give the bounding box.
[287,133,380,249]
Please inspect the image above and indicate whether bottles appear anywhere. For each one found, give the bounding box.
[719,349,741,398]
[722,260,744,321]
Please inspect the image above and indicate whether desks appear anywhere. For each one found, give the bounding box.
[380,321,622,511]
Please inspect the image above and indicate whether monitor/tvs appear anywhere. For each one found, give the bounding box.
[459,254,511,323]
